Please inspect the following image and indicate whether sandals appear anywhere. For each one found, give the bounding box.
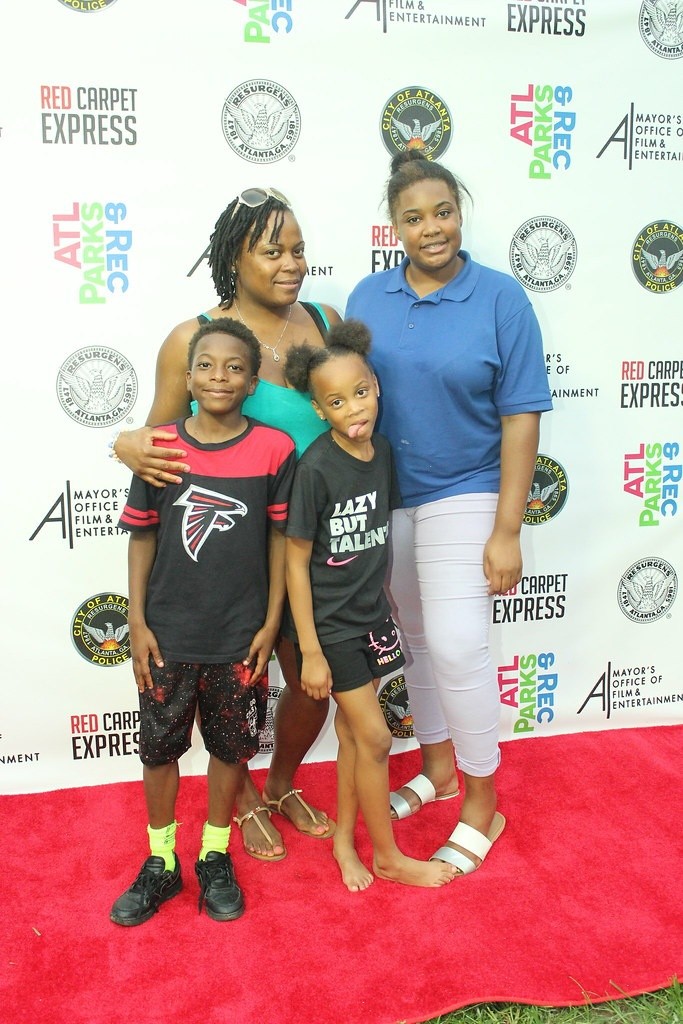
[233,806,288,862]
[261,788,337,839]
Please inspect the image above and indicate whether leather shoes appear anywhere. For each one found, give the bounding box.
[194,850,244,921]
[109,851,183,927]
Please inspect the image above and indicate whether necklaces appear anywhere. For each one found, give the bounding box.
[233,296,292,362]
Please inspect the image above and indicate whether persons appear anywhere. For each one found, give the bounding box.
[110,187,344,861]
[284,319,457,892]
[109,318,297,927]
[345,149,553,876]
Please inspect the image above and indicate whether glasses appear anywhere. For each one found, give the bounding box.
[231,186,294,220]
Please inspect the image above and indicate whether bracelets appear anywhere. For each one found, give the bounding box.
[111,430,129,463]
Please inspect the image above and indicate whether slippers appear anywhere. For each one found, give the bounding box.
[389,773,460,821]
[427,810,507,877]
